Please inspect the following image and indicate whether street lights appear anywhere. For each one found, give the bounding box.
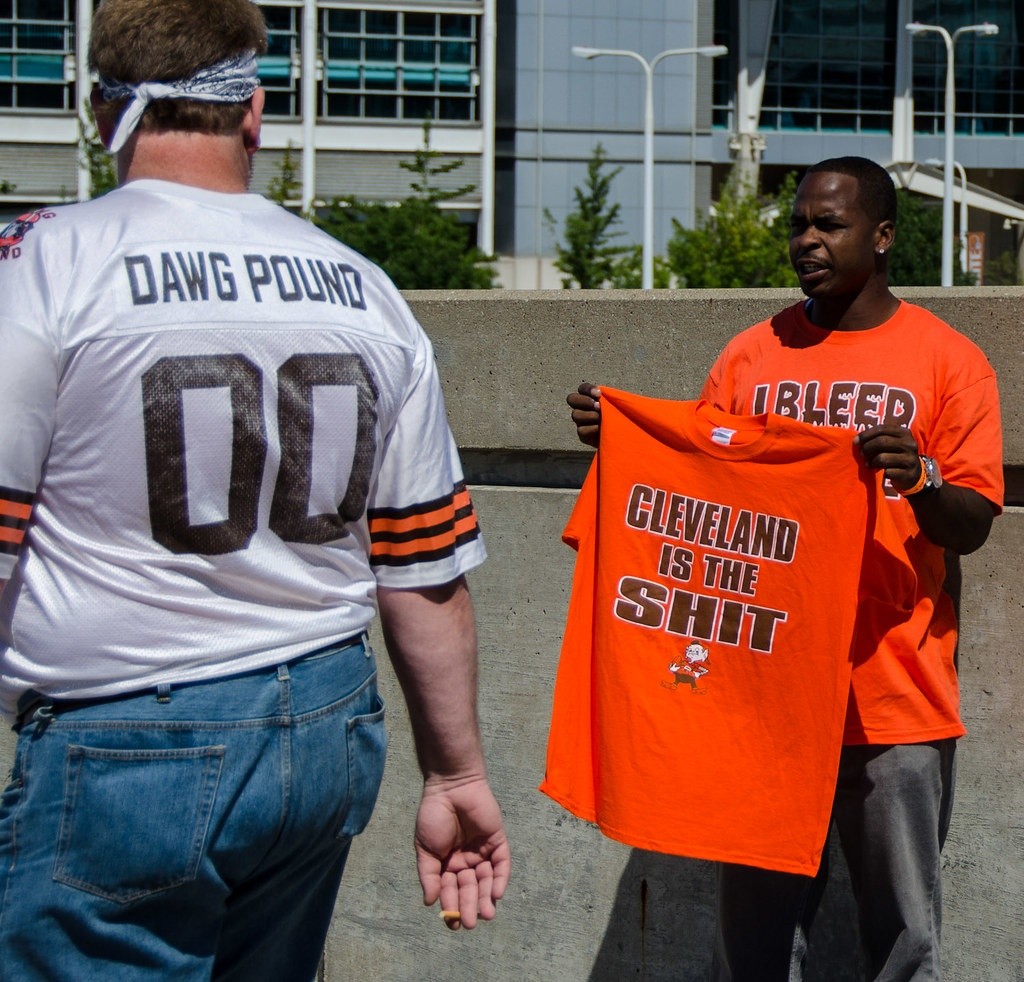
[904,23,1004,288]
[925,160,968,283]
[570,45,729,289]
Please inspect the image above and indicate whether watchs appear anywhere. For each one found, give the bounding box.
[903,454,943,502]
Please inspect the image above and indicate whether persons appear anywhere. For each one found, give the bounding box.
[0,0,513,979]
[564,155,1006,981]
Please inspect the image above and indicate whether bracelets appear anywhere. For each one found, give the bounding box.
[889,453,928,497]
